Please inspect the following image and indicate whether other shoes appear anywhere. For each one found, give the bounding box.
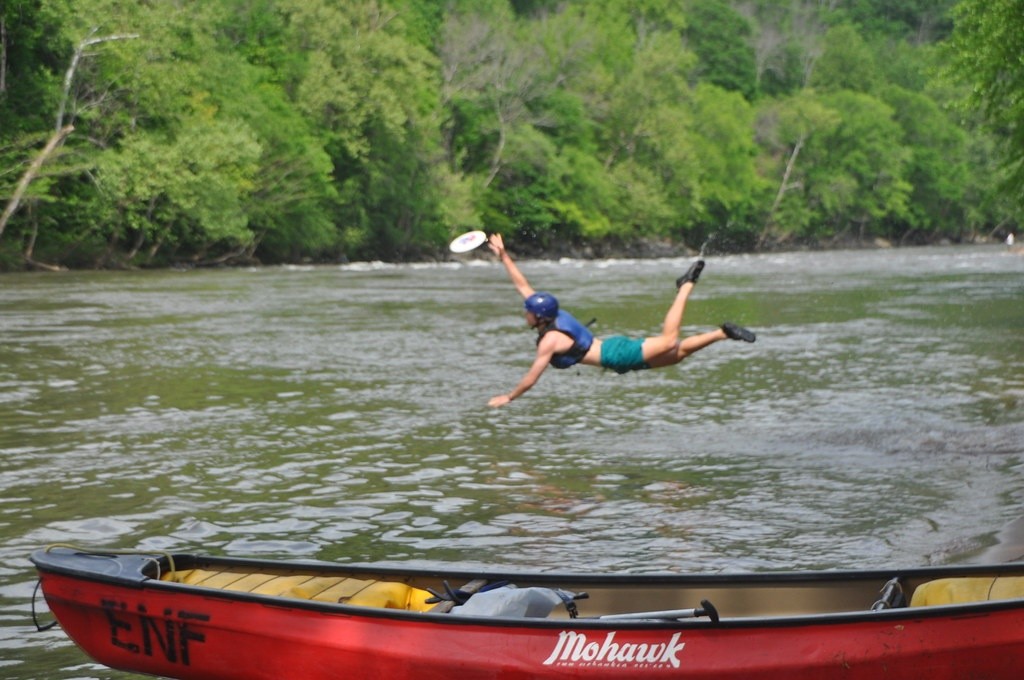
[721,321,756,344]
[676,260,706,290]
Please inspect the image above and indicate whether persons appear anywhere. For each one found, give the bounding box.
[488,233,756,409]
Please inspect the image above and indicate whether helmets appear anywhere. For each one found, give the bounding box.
[522,290,561,318]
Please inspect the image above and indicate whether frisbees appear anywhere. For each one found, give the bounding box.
[449,230,486,254]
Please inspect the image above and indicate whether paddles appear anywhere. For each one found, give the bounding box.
[576,598,722,629]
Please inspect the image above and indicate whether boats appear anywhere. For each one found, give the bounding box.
[29,543,1024,680]
